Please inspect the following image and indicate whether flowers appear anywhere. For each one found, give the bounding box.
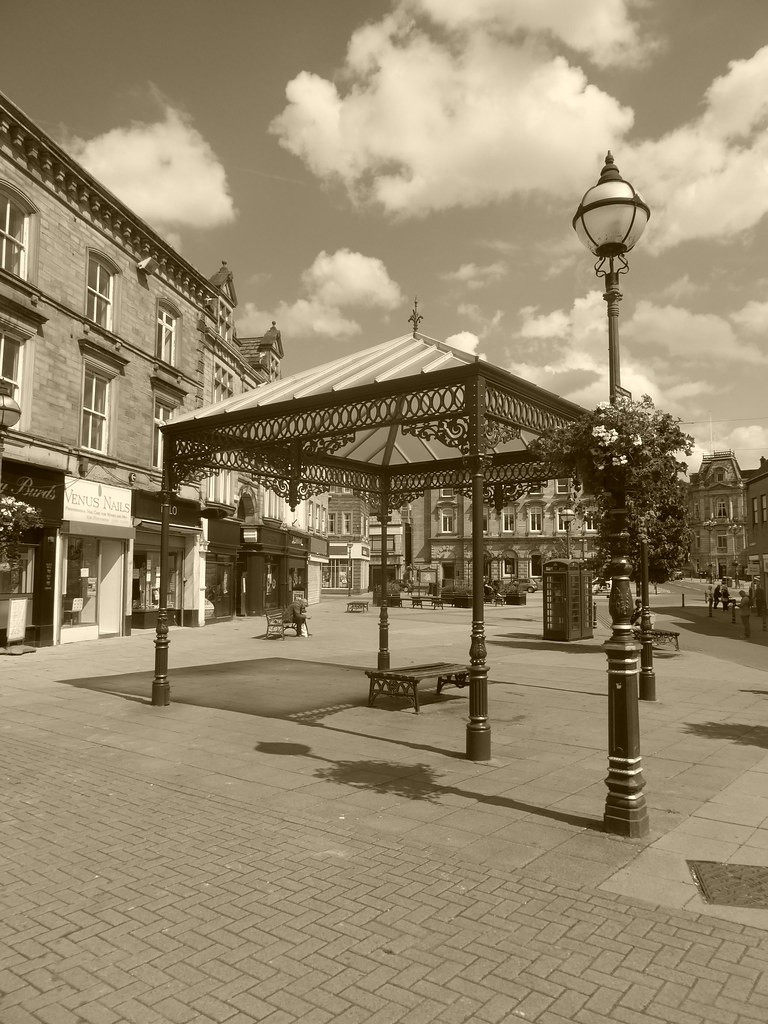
[546,395,690,487]
[0,494,44,534]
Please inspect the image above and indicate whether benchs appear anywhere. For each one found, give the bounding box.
[265,605,312,640]
[393,595,507,607]
[346,601,369,614]
[634,629,682,651]
[408,589,427,595]
[367,660,473,714]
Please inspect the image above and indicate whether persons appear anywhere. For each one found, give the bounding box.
[720,588,730,611]
[630,599,643,625]
[737,590,751,638]
[631,608,654,631]
[752,585,763,617]
[282,599,311,637]
[706,586,713,601]
[713,585,721,609]
